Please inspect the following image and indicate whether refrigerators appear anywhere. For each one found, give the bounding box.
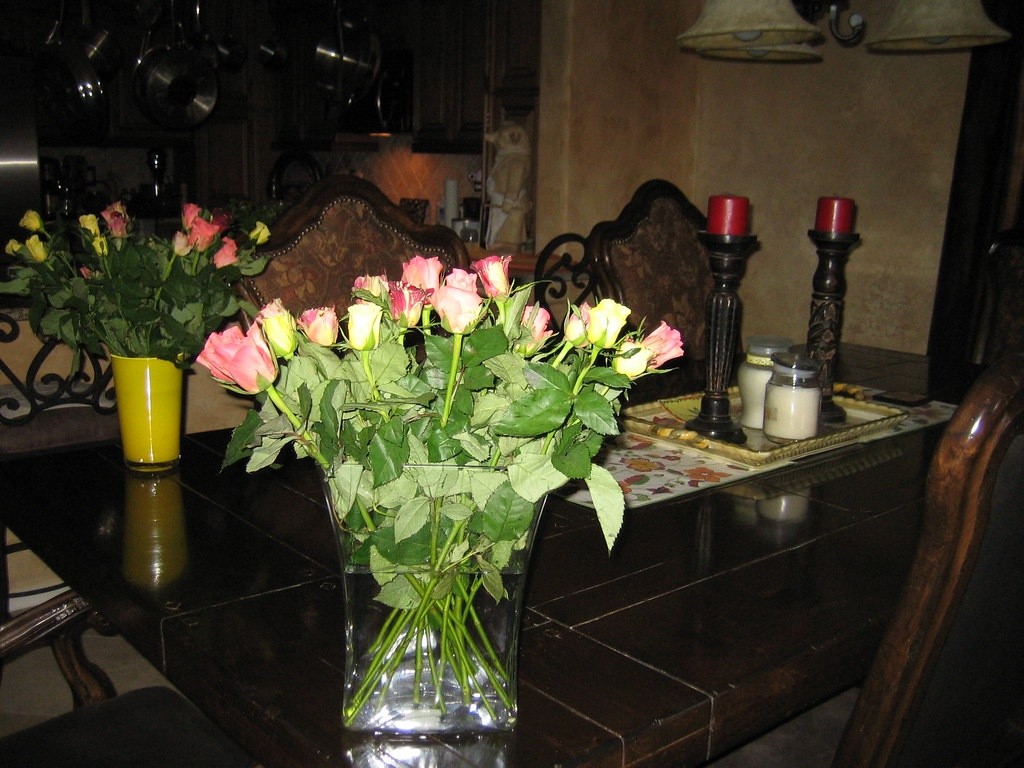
[0,51,43,306]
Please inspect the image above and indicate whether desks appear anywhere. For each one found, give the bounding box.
[0,341,992,767]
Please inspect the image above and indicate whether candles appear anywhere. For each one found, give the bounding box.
[708,192,750,236]
[815,192,855,235]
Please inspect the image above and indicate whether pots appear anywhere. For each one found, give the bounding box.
[315,0,382,113]
[132,0,221,132]
[40,0,124,143]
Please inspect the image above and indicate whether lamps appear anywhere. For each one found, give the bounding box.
[676,0,1010,63]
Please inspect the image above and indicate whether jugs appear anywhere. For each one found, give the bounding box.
[63,154,97,192]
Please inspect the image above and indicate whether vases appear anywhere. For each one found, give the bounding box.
[109,353,181,472]
[317,463,547,733]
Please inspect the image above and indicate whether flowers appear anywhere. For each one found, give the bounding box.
[193,254,686,726]
[0,203,272,369]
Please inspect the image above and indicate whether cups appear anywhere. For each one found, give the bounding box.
[738,335,793,430]
[763,354,822,443]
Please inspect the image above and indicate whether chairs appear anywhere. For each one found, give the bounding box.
[963,228,1024,364]
[536,175,760,369]
[220,175,469,337]
[708,362,1024,768]
[1,295,128,711]
